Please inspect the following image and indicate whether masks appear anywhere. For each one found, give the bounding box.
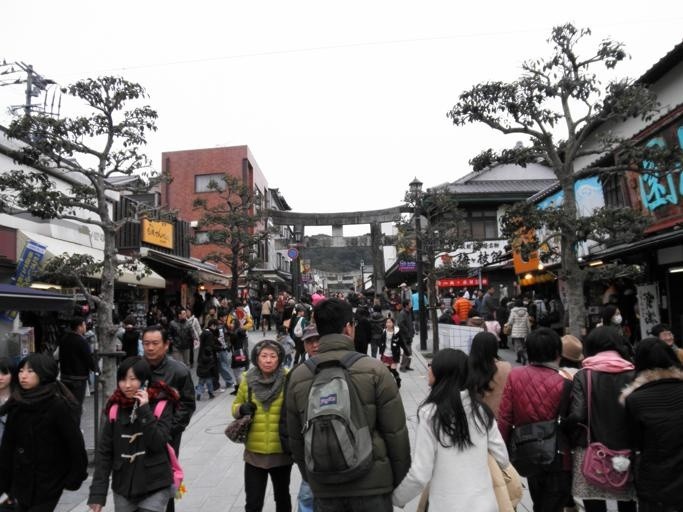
[612,315,622,324]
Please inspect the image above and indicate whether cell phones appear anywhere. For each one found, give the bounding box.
[140,380,149,391]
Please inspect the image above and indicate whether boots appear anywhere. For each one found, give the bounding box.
[516,352,521,362]
[518,351,527,365]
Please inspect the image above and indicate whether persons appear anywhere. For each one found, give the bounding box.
[61,279,682,511]
[2,351,89,511]
[0,358,18,445]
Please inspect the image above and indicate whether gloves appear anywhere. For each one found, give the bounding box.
[240,402,257,415]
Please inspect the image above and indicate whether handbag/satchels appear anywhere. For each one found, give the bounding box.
[503,325,511,334]
[225,415,253,443]
[233,349,247,362]
[581,442,634,492]
[293,316,304,337]
[509,420,558,478]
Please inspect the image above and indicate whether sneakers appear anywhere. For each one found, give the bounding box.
[400,368,414,372]
[196,382,239,400]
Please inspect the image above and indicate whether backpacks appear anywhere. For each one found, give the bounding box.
[302,352,376,485]
[106,400,185,497]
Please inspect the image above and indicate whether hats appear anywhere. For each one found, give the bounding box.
[561,334,585,361]
[301,323,320,341]
[251,339,285,367]
